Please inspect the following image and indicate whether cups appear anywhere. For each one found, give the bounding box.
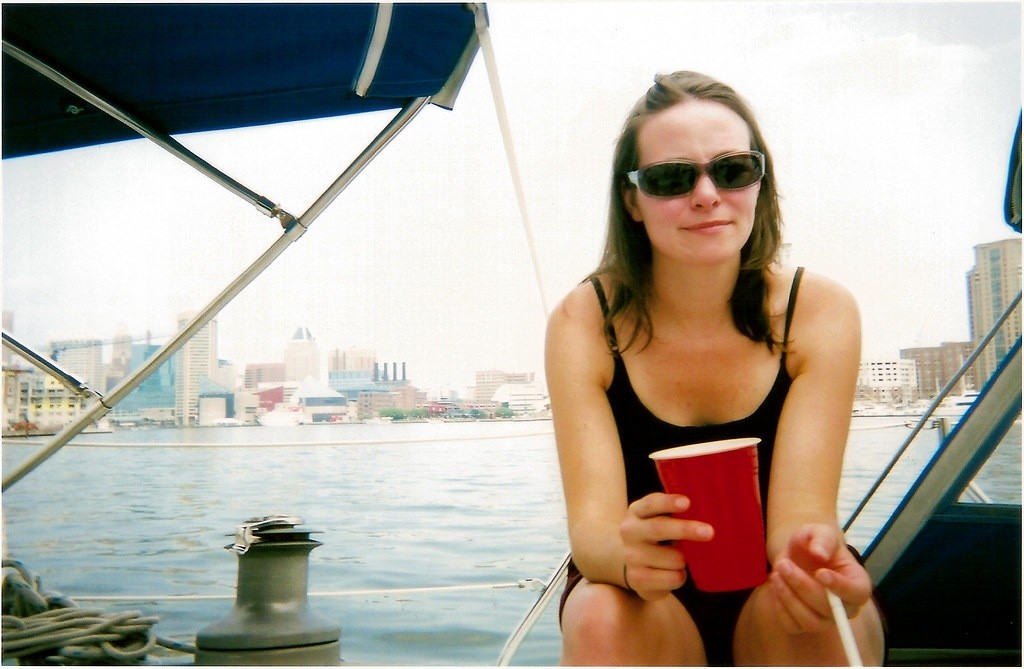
[649,437,767,593]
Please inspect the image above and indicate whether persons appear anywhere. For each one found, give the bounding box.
[544,73,885,666]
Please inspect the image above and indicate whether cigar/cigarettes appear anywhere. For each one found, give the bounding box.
[825,588,863,667]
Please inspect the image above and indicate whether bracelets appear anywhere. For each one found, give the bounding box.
[623,562,632,589]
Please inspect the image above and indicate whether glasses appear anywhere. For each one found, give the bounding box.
[624,151,766,198]
